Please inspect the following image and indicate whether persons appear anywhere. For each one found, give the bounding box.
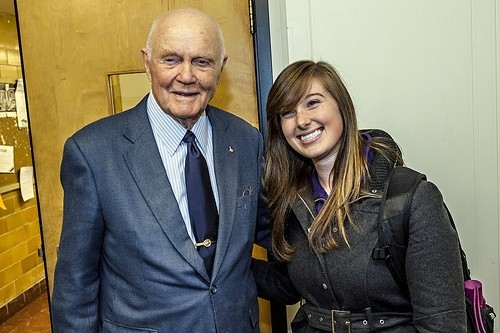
[51,7,469,333]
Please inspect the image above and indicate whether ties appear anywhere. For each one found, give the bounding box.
[183,130,219,283]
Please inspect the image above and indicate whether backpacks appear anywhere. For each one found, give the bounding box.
[376,169,495,333]
